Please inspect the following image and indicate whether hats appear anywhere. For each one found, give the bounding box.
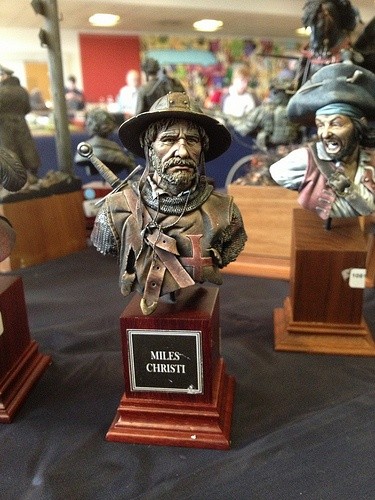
[143,58,160,72]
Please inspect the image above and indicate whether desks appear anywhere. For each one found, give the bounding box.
[30,125,258,188]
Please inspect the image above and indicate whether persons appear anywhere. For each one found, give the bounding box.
[90,92,248,315]
[134,57,161,116]
[222,68,260,116]
[74,107,127,166]
[28,88,48,116]
[63,76,85,111]
[117,70,141,115]
[270,63,375,219]
[0,64,41,177]
